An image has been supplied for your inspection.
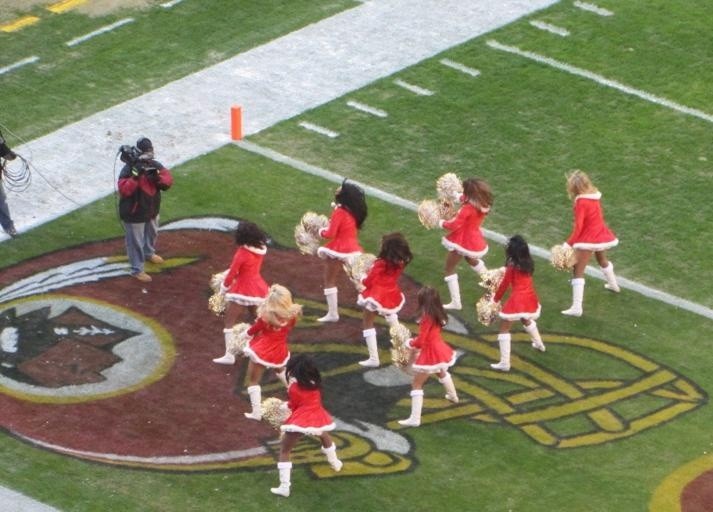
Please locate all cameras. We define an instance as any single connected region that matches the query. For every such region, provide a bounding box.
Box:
[119,144,161,181]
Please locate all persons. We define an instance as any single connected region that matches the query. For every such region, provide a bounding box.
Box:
[233,283,304,422]
[418,171,500,309]
[390,283,462,426]
[263,351,344,499]
[293,177,369,323]
[0,127,18,241]
[478,234,547,372]
[350,230,414,368]
[206,219,276,366]
[114,138,174,284]
[549,168,621,317]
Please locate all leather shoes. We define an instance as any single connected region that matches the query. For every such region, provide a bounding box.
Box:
[131,271,152,282]
[146,254,164,263]
[4,222,16,237]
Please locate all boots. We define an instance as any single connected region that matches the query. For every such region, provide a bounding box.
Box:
[491,334,511,371]
[386,314,400,331]
[399,390,423,427]
[441,273,463,308]
[351,273,368,294]
[244,385,262,421]
[471,259,488,277]
[321,442,343,472]
[523,320,545,352]
[276,367,291,393]
[438,372,458,403]
[318,288,339,322]
[600,262,620,293]
[213,329,235,365]
[359,328,378,366]
[561,278,585,317]
[271,462,292,497]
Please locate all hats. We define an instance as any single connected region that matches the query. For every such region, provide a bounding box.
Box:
[136,137,153,149]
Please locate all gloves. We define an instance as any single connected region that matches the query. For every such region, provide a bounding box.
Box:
[132,163,142,177]
[145,167,160,179]
